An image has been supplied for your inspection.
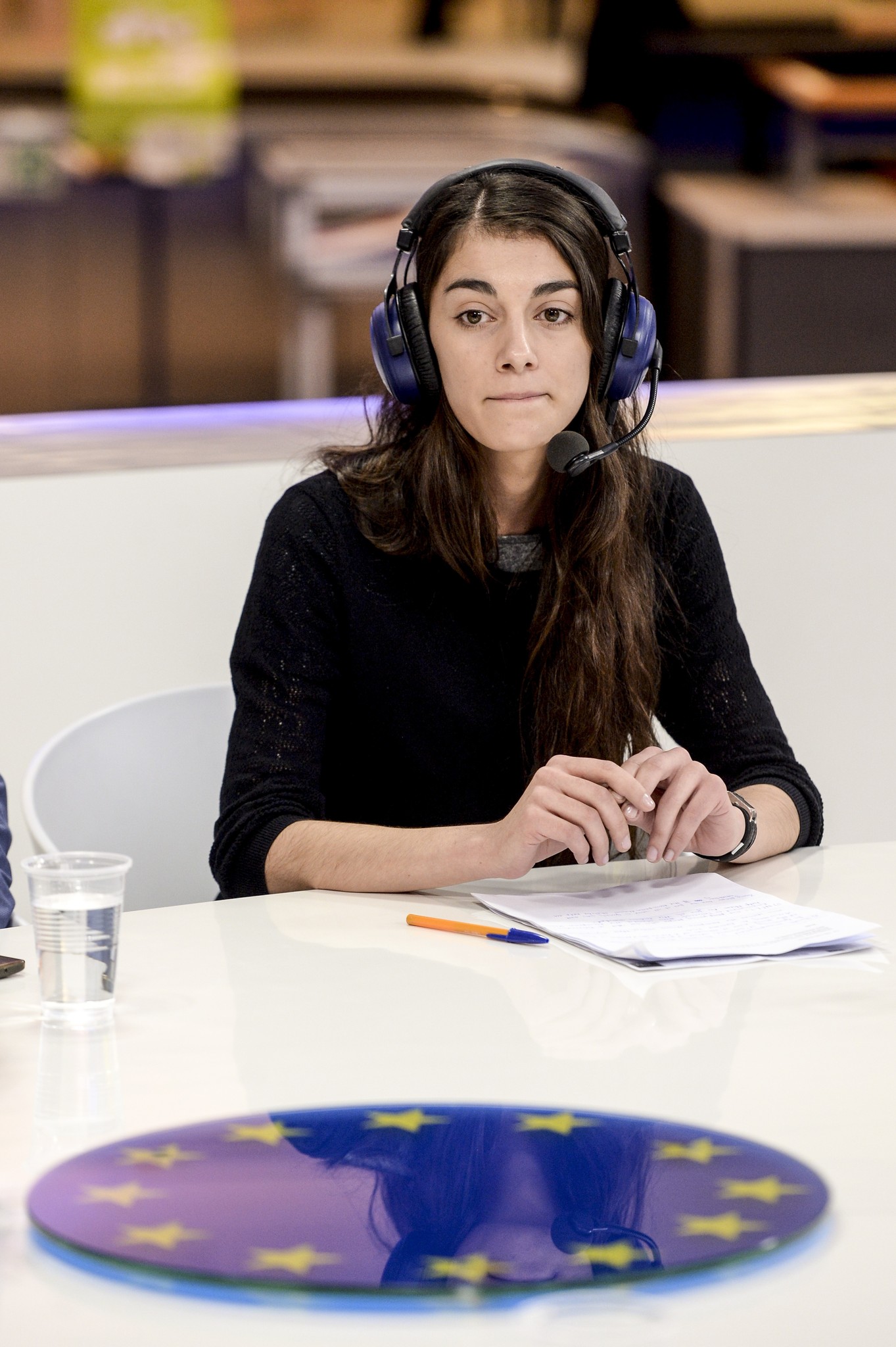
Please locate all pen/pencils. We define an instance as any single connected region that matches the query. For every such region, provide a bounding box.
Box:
[406,913,549,945]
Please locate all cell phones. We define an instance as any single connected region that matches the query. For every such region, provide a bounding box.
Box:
[0,955,25,978]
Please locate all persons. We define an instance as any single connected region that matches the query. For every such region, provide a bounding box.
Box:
[209,159,824,902]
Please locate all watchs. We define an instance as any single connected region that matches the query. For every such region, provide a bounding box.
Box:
[692,791,757,862]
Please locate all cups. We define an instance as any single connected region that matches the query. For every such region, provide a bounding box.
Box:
[19,851,133,1028]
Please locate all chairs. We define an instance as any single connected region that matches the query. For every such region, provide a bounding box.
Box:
[22,680,249,901]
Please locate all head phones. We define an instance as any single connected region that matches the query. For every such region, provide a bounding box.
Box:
[368,161,658,407]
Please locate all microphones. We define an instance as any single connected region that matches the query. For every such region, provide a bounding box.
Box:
[547,340,664,475]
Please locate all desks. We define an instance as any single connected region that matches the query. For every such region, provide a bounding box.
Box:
[660,169,894,381]
[0,839,895,1347]
